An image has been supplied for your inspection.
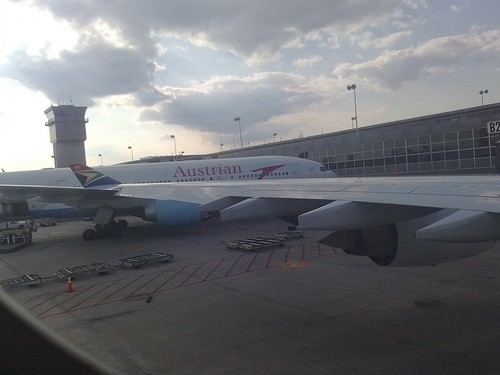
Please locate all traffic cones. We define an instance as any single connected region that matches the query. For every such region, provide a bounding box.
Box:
[65,276,73,293]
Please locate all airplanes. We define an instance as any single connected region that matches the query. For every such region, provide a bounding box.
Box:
[0,155,500,269]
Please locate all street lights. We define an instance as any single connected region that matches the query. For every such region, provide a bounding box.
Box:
[99,154,103,165]
[234,117,242,147]
[347,83,358,128]
[479,89,488,105]
[128,146,134,161]
[351,117,356,129]
[273,133,277,142]
[170,135,176,155]
